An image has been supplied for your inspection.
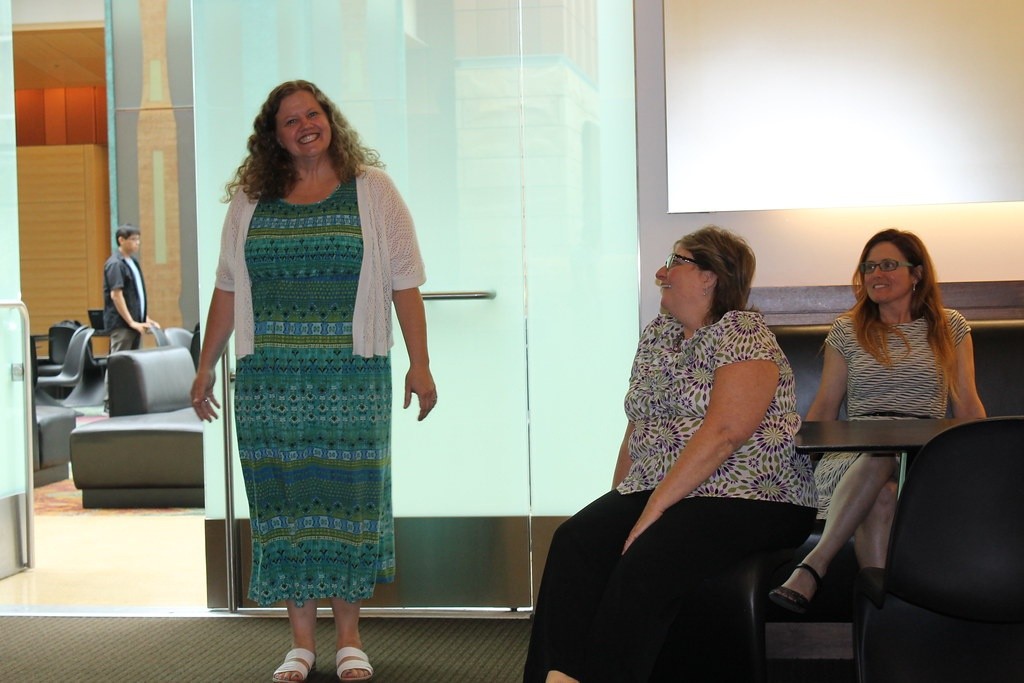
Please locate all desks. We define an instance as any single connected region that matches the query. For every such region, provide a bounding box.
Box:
[794,416,1024,500]
[31,330,110,359]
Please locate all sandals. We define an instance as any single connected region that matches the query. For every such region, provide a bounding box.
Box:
[769,564,823,614]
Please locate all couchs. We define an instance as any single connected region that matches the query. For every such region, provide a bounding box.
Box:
[33,347,205,508]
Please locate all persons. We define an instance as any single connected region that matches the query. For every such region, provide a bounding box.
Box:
[771,228,987,615]
[104,223,160,414]
[521,228,818,683]
[190,80,437,683]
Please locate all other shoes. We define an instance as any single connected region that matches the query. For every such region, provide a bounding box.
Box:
[104,401,109,412]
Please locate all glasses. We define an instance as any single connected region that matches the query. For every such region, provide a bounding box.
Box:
[665,253,697,268]
[859,259,913,274]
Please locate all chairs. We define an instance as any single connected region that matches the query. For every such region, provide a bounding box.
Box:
[29,320,200,415]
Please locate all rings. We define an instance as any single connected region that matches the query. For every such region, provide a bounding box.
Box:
[205,400,208,402]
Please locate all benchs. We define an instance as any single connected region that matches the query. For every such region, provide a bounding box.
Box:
[723,321,1024,682]
[841,415,1024,682]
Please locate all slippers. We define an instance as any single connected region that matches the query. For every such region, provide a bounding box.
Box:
[335,647,374,683]
[272,648,316,683]
